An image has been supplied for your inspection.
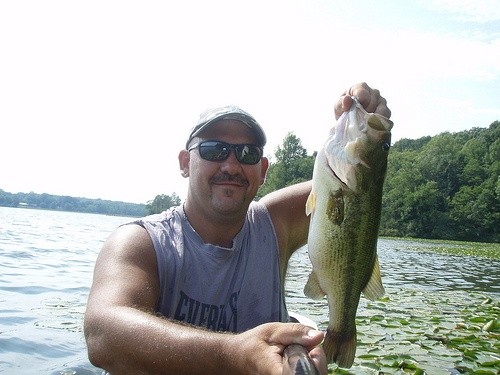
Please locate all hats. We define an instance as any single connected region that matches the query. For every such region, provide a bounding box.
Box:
[185,105,266,150]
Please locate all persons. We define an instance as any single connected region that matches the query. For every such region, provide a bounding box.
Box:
[84,82,391,375]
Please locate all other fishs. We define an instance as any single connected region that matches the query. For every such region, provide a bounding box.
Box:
[303,96,393,368]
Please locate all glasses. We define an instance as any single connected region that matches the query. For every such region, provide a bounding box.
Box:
[189,139,262,165]
